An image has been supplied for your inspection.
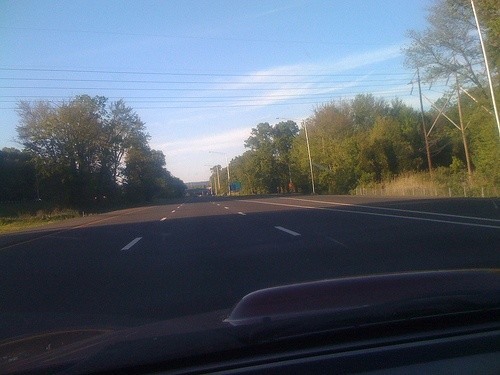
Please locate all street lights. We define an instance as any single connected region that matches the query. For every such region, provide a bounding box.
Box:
[209,151,232,197]
[276,118,316,195]
